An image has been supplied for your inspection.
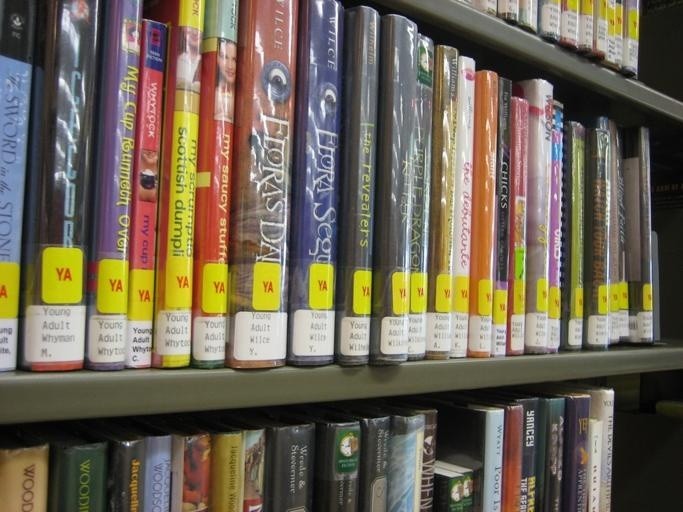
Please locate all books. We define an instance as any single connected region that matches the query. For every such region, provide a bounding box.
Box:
[2,384,615,512]
[1,0,658,374]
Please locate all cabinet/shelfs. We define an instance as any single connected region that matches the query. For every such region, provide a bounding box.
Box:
[0,1,683,512]
